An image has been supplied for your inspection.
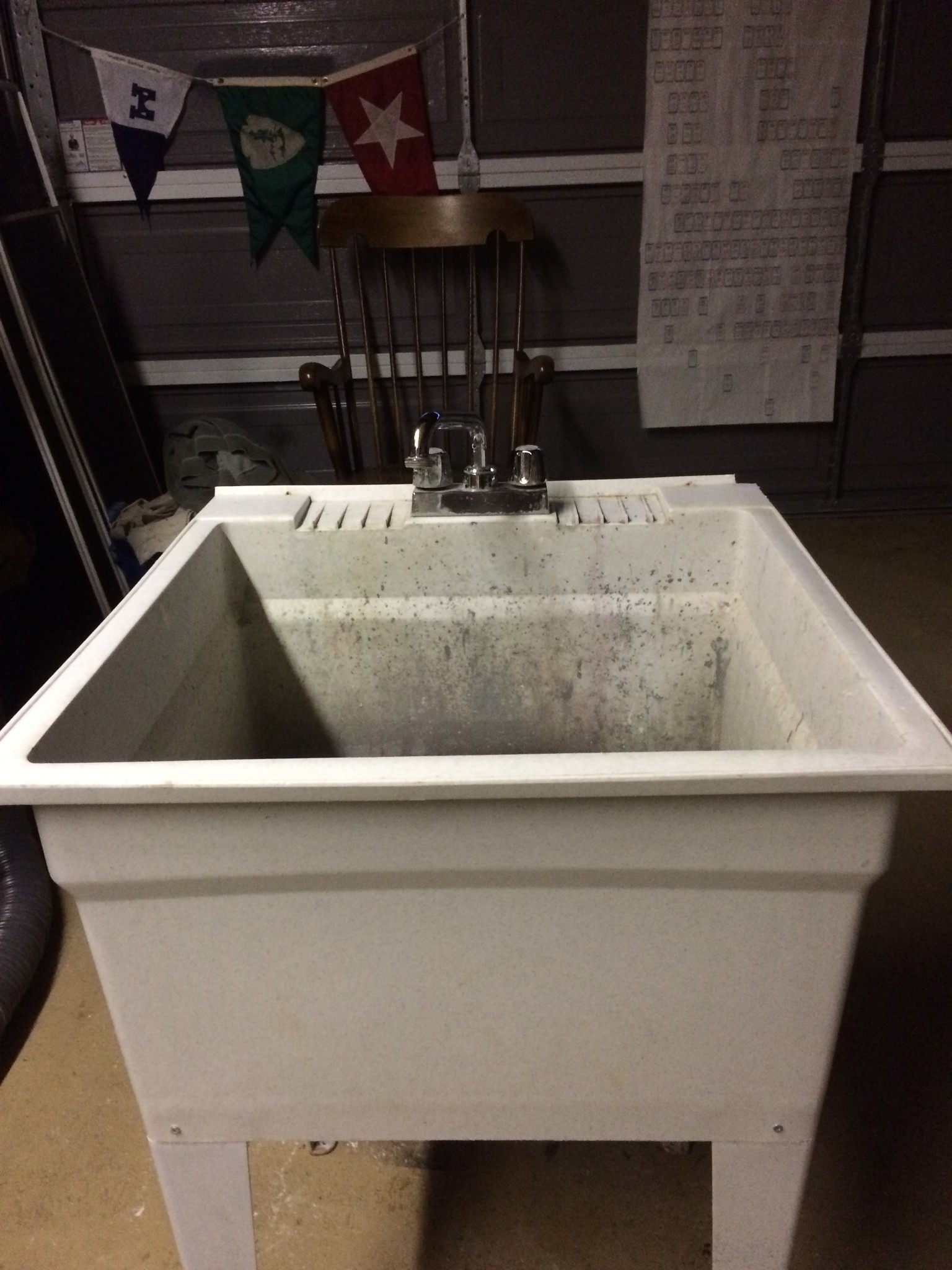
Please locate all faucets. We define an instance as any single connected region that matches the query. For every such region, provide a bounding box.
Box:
[405,410,554,518]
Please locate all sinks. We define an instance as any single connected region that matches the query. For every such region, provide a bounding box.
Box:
[1,477,952,1150]
